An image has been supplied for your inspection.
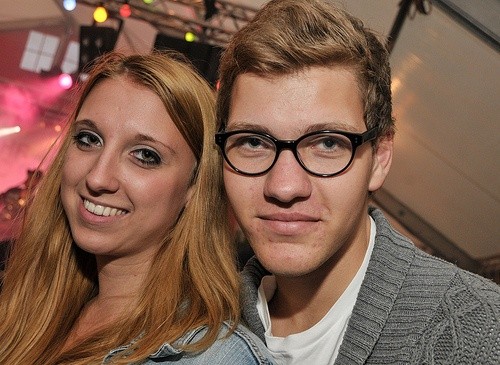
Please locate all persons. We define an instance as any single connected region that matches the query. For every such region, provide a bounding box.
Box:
[214,0,500,365]
[0,52,278,365]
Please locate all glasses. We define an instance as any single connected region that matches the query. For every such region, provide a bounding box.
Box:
[215,121,379,177]
